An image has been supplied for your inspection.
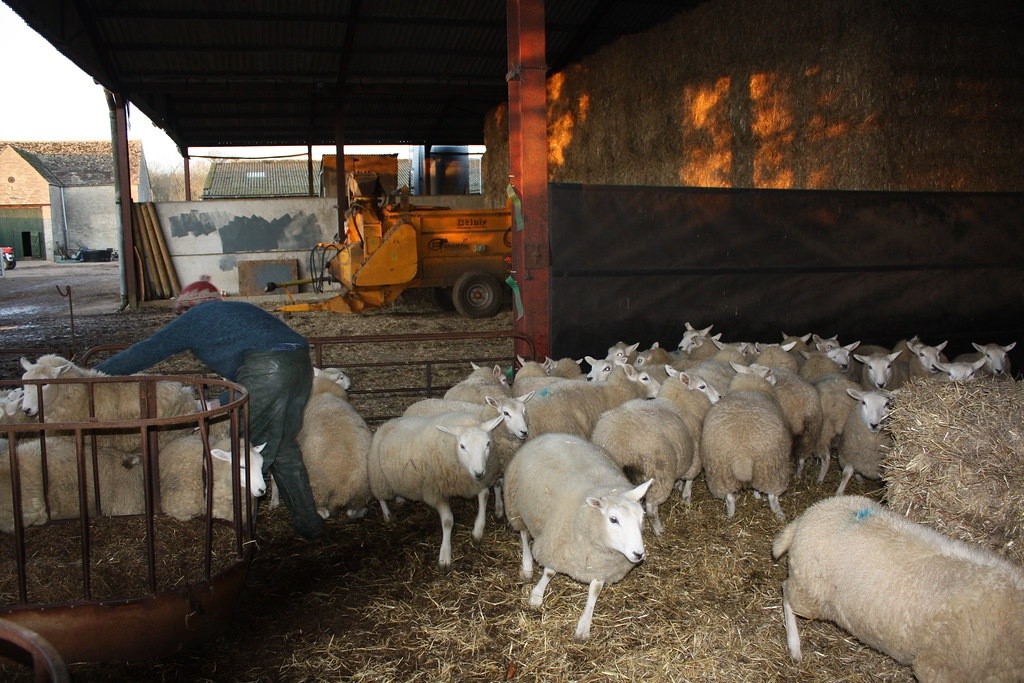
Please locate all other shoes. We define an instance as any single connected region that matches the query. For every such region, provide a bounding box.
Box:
[304,522,326,539]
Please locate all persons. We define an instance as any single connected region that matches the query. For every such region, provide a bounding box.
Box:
[88,281,325,537]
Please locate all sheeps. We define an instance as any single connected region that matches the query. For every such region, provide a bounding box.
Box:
[771,493,1023,682]
[0,320,1023,645]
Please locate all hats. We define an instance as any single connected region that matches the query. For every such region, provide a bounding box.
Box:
[175,280,221,316]
